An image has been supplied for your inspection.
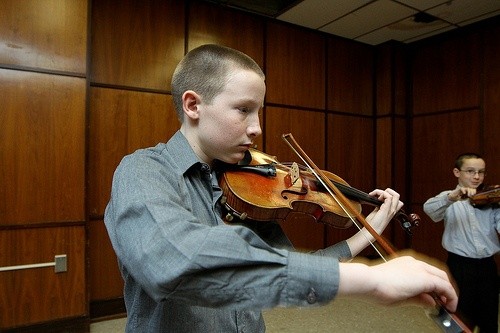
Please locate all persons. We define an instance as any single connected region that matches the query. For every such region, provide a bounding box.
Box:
[103,43,460,333]
[422,152,500,333]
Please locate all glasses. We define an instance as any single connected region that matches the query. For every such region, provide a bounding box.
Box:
[459,169,487,176]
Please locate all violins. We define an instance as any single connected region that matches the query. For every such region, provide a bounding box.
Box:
[470,184,500,206]
[218,147,421,233]
